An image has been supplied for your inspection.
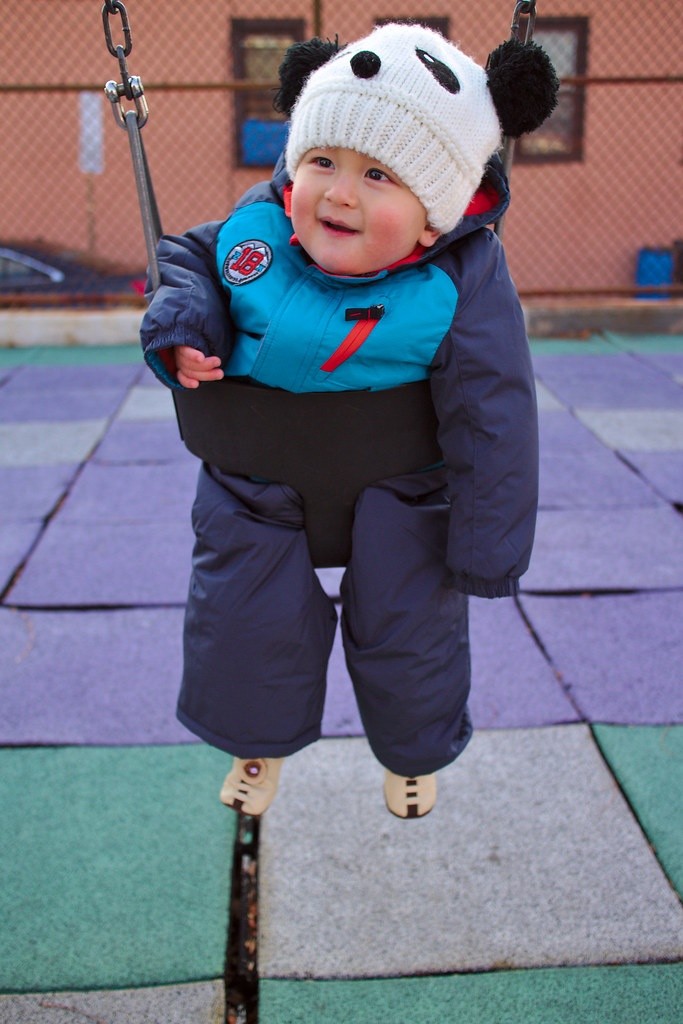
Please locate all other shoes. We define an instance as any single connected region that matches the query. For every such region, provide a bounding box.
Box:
[381,768,437,818]
[219,753,284,816]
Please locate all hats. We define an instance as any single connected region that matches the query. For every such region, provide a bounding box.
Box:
[272,20,562,233]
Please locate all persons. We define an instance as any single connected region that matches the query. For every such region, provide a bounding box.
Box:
[139,24,559,819]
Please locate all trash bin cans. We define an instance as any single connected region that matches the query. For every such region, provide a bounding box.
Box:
[635,247,673,300]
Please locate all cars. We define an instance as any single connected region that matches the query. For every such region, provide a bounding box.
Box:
[0,242,148,298]
